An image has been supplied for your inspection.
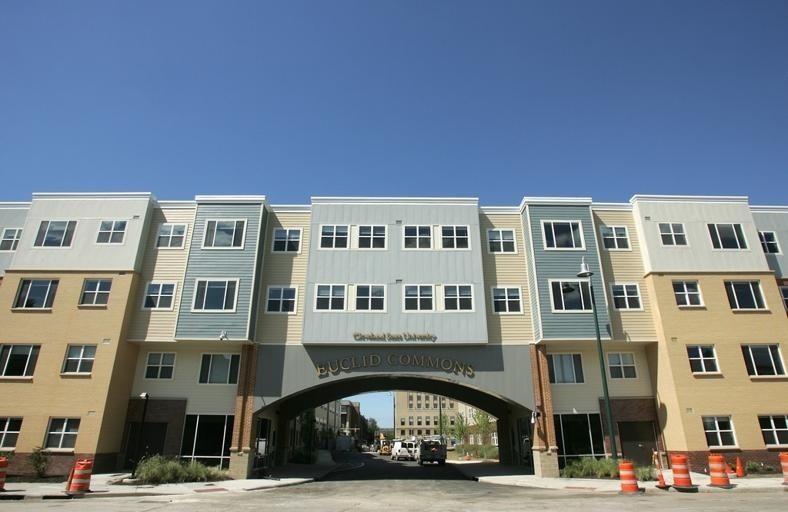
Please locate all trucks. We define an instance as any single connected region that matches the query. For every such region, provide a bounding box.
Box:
[372,437,447,466]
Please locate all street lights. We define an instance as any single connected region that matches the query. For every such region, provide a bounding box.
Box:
[574,251,617,468]
[129,392,149,480]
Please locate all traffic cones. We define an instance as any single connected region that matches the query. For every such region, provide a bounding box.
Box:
[733,457,746,479]
